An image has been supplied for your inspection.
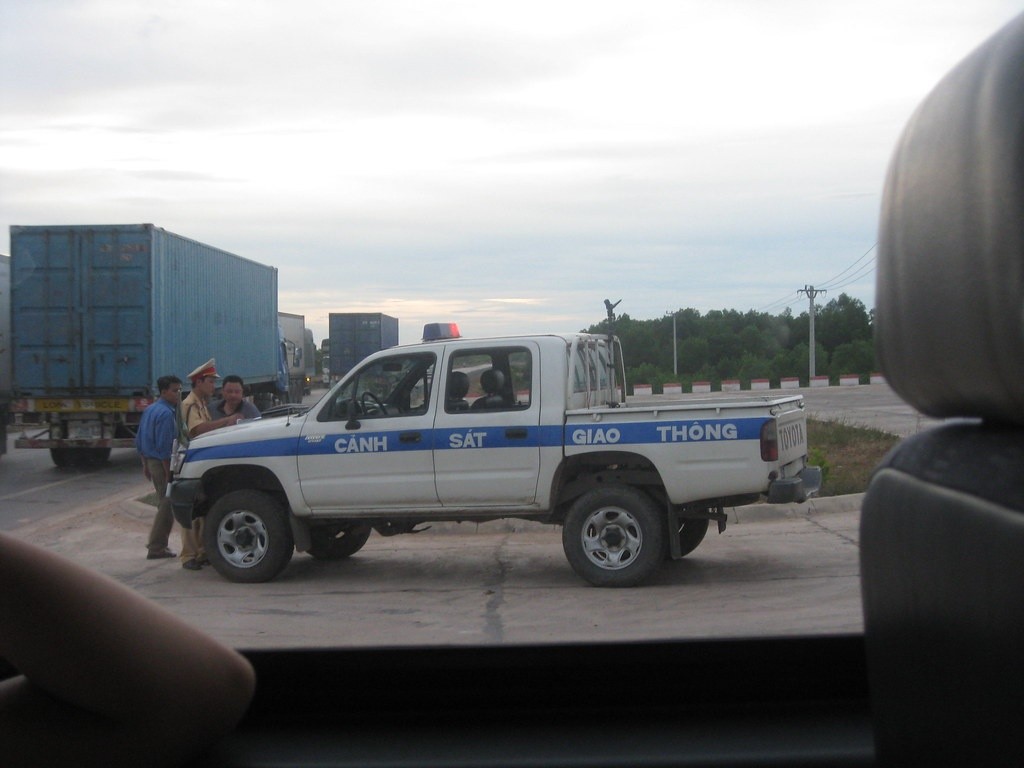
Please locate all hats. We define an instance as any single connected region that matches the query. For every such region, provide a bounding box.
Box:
[187,358,221,383]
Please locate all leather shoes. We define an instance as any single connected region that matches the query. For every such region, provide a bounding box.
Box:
[147,548,177,559]
[183,559,208,570]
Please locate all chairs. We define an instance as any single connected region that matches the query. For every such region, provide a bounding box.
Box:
[471,369,505,409]
[857,8,1024,768]
[447,370,470,412]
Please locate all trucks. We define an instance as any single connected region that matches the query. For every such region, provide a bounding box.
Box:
[9,223,303,472]
[0,254,14,454]
[276,311,317,396]
[328,312,400,391]
[320,338,345,388]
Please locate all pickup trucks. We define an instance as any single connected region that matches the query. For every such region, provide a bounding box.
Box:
[167,319,821,588]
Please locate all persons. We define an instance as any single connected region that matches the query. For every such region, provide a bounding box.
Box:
[208,373,262,424]
[133,374,184,559]
[173,356,227,570]
